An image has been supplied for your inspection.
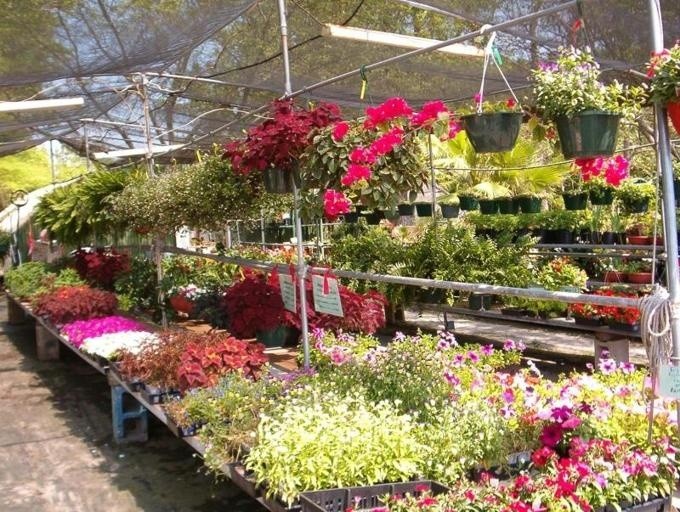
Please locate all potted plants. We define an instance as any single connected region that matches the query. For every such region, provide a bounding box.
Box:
[225,278,290,347]
[601,258,626,282]
[479,197,498,214]
[562,189,588,210]
[103,177,158,233]
[617,183,658,214]
[590,176,616,206]
[415,201,433,217]
[398,200,415,216]
[498,194,518,214]
[162,267,216,316]
[221,95,340,192]
[471,214,625,245]
[627,259,652,284]
[457,189,480,210]
[227,405,418,512]
[513,191,548,213]
[468,270,496,311]
[627,223,650,244]
[438,199,459,218]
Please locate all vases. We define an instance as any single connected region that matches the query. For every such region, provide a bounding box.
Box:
[666,94,680,136]
[459,109,522,155]
[608,318,640,331]
[571,313,605,326]
[550,109,623,158]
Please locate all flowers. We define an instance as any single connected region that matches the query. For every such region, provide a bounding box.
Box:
[471,94,523,113]
[607,291,641,324]
[571,291,606,319]
[530,44,621,117]
[639,39,680,105]
